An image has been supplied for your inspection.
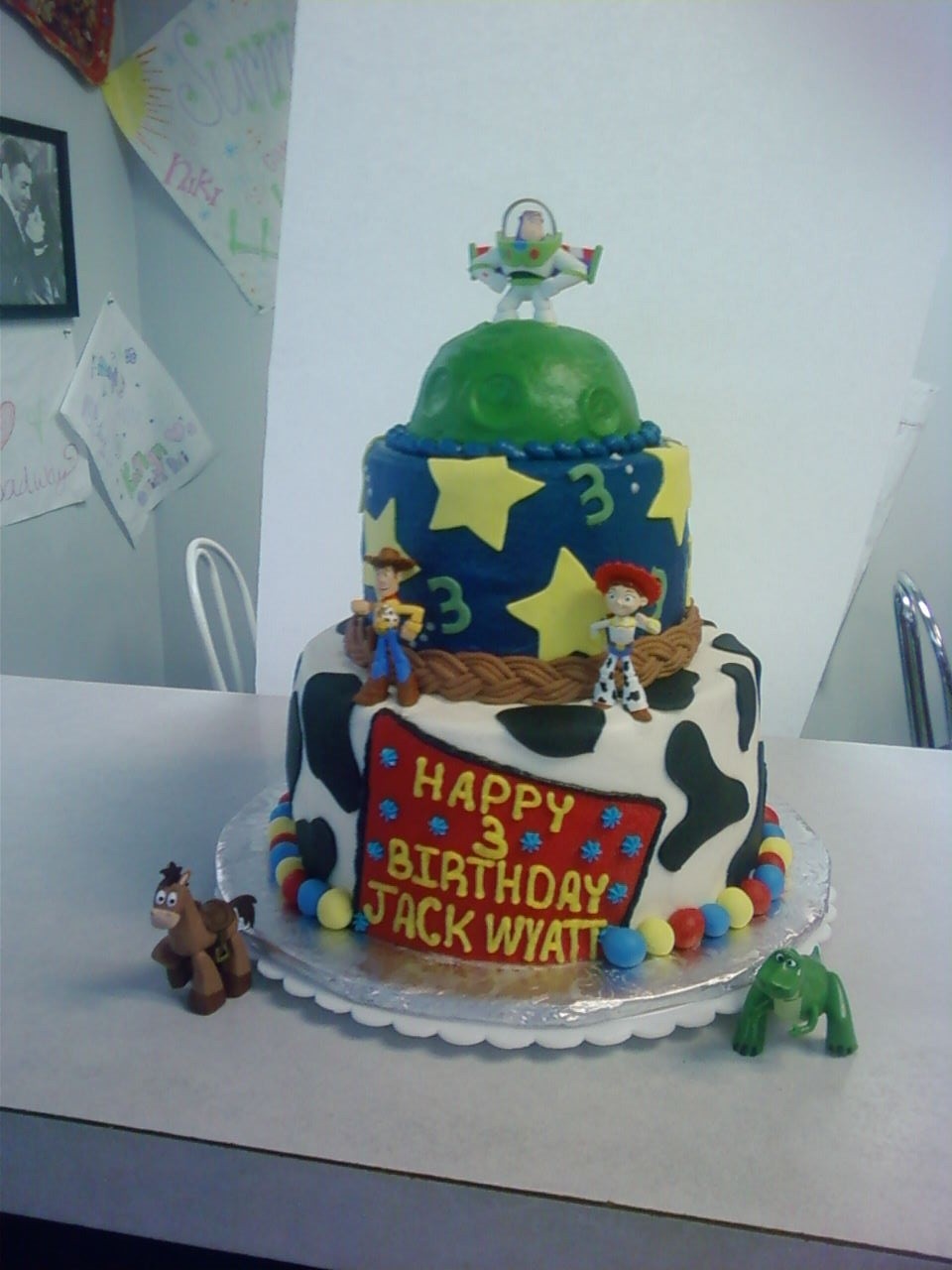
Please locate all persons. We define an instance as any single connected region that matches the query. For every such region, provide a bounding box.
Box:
[350,546,424,705]
[0,136,68,306]
[468,198,604,322]
[588,562,662,722]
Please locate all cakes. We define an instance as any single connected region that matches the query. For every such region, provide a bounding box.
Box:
[261,199,794,967]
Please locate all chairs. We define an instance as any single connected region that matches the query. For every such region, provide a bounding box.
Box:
[890,568,952,756]
[184,535,256,691]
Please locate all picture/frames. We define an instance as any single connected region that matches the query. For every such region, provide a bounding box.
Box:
[0,114,82,321]
